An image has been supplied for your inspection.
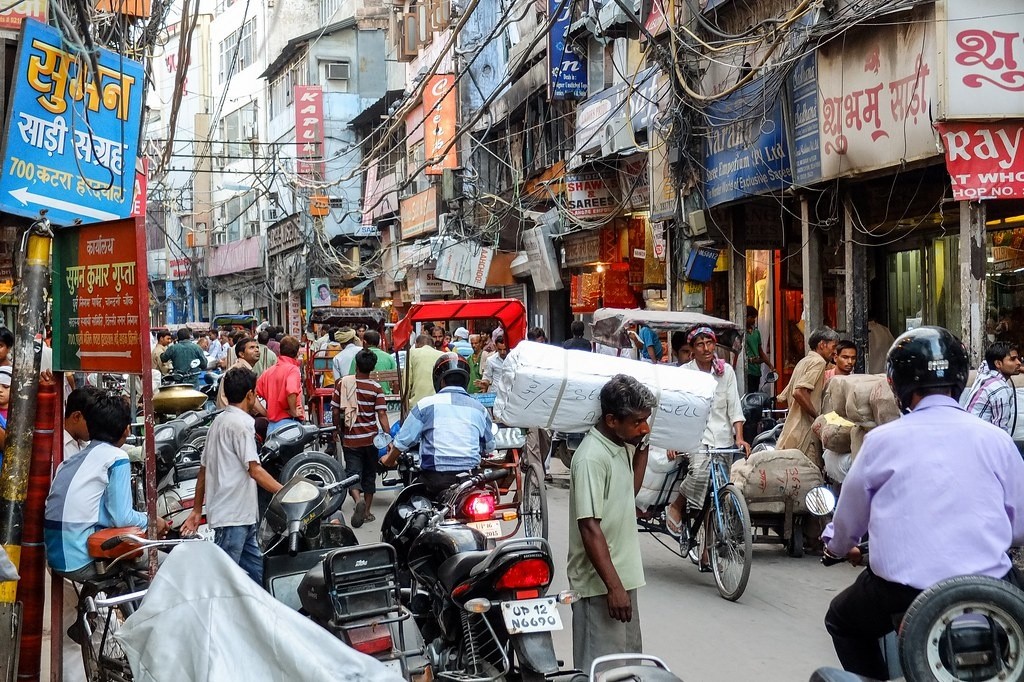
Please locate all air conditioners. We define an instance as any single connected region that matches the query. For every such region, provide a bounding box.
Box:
[599,117,635,156]
[216,157,224,169]
[325,63,350,80]
[218,233,226,245]
[243,125,253,139]
[245,205,257,223]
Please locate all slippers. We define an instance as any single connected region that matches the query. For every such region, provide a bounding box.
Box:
[701,561,714,572]
[664,505,684,537]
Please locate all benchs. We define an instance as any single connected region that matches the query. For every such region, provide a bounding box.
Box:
[307,349,343,371]
[368,369,403,400]
[308,369,334,404]
[468,392,518,428]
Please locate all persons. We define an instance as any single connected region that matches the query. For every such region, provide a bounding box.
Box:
[819,325,1024,681]
[559,306,860,573]
[159,317,306,442]
[0,326,171,647]
[865,310,896,375]
[963,341,1022,435]
[379,318,553,496]
[567,373,657,677]
[178,367,283,585]
[297,323,403,528]
[317,284,331,304]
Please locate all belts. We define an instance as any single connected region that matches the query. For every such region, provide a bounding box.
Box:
[284,416,303,424]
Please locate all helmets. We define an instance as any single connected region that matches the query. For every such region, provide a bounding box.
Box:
[432,351,471,393]
[884,324,969,414]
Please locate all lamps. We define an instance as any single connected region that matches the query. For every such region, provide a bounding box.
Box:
[212,218,222,231]
[262,209,278,222]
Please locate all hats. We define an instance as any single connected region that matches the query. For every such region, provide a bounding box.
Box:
[0,366,12,386]
[335,326,357,343]
[454,327,469,339]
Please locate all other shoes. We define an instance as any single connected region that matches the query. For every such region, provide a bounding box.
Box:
[363,512,376,523]
[350,502,366,528]
[804,545,826,556]
[67,623,80,645]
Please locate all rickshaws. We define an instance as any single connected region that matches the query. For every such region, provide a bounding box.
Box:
[517,422,755,602]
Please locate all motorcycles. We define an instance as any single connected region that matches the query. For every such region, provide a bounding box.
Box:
[800,486,1023,682]
[64,306,792,682]
